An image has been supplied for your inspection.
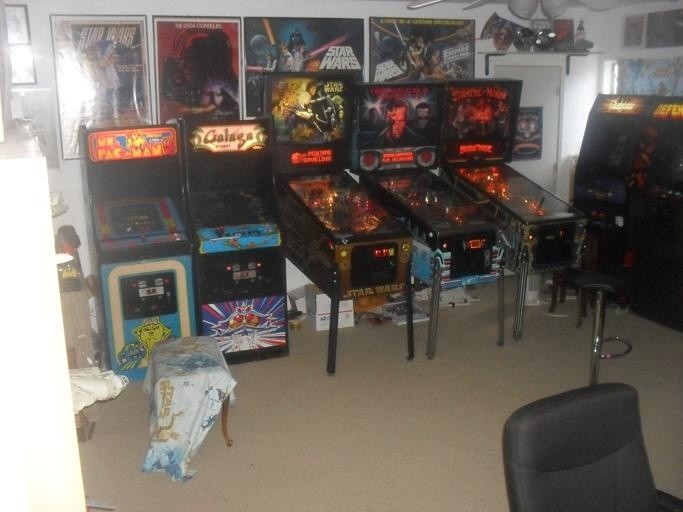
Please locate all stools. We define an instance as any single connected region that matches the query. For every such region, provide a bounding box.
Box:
[562,267,638,384]
[151,335,233,450]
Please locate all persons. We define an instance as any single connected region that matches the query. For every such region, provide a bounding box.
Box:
[363,99,436,149]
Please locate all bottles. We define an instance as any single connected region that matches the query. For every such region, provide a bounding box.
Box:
[574,19,588,51]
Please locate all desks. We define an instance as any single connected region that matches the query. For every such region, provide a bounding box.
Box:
[58,251,96,370]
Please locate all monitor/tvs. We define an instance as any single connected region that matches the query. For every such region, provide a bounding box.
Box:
[583,123,635,178]
[198,192,260,228]
[108,204,164,235]
[662,126,683,190]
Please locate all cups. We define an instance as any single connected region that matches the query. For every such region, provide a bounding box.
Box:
[48,191,61,206]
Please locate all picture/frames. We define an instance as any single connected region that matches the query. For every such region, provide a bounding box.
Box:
[4,3,32,46]
[50,14,152,162]
[9,46,37,85]
[152,15,243,126]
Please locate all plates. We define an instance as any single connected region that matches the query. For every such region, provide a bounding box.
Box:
[532,27,556,48]
[56,253,74,263]
[512,27,536,51]
[491,26,512,51]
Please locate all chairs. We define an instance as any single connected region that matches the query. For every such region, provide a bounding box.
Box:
[502,382,683,511]
[549,226,602,327]
